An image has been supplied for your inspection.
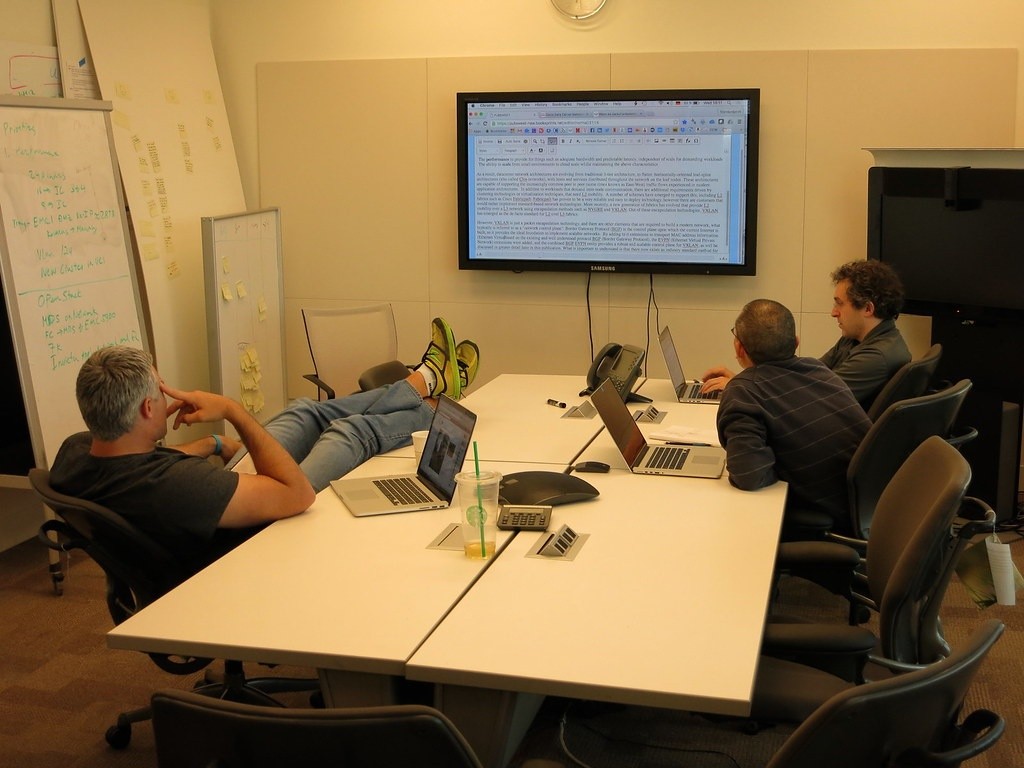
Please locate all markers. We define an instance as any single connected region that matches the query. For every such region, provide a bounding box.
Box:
[547,398,566,408]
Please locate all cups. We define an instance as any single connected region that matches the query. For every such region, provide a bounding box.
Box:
[411,431,430,465]
[454,471,503,559]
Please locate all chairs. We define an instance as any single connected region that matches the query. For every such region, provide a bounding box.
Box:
[554,615,1006,768]
[790,379,975,532]
[300,303,415,409]
[146,686,486,768]
[863,343,944,422]
[765,435,996,682]
[26,463,326,748]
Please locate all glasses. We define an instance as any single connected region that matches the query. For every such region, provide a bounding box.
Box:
[732,327,749,355]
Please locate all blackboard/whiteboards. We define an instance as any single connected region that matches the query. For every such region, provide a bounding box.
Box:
[0,96,147,472]
[201,206,287,442]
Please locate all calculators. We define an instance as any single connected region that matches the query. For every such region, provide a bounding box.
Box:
[497,503,554,530]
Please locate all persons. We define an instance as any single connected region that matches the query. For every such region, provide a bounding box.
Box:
[701,257,911,414]
[717,299,873,532]
[49,318,479,601]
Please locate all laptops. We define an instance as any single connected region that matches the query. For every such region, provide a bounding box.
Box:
[591,377,726,478]
[330,393,477,517]
[657,325,724,404]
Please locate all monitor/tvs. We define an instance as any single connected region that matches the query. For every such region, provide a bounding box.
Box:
[455,87,760,276]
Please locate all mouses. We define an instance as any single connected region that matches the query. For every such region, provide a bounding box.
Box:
[576,461,610,473]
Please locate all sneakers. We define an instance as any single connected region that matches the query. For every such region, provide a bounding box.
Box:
[453,340,480,400]
[422,317,461,403]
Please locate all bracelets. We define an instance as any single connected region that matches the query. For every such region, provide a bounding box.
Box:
[213,435,222,455]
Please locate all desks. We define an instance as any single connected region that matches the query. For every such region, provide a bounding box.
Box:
[102,368,791,768]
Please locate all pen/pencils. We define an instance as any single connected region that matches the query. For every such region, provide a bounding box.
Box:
[665,438,718,447]
